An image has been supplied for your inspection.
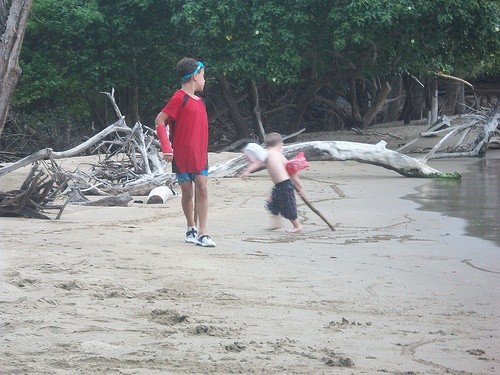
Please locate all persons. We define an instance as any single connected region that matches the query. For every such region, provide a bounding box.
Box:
[240,132,306,234]
[155,58,217,247]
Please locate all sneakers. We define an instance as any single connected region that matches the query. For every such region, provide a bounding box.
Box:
[185,226,198,242]
[197,234,217,246]
